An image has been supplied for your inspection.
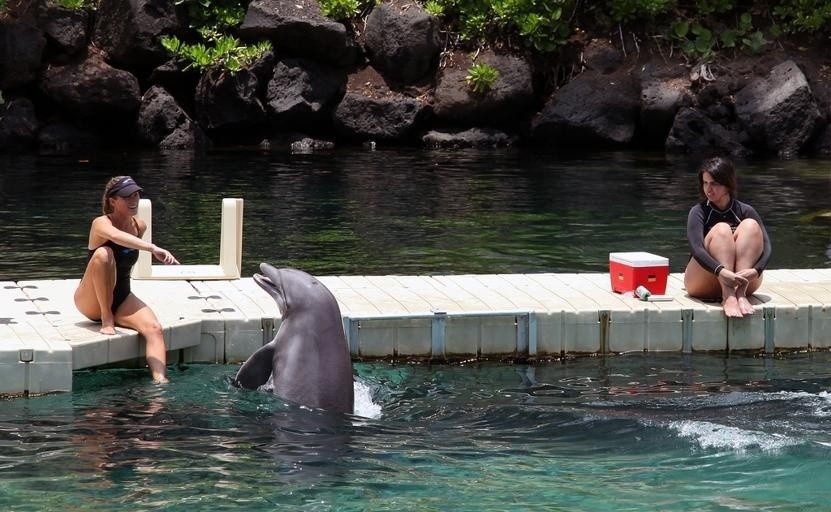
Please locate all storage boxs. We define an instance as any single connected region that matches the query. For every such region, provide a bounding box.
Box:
[608,248,671,295]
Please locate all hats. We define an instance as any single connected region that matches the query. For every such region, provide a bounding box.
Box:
[107,178,143,197]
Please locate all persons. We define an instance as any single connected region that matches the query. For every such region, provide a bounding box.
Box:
[74,175,181,383]
[685,158,772,319]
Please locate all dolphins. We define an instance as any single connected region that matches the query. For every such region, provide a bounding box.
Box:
[233,260,354,420]
